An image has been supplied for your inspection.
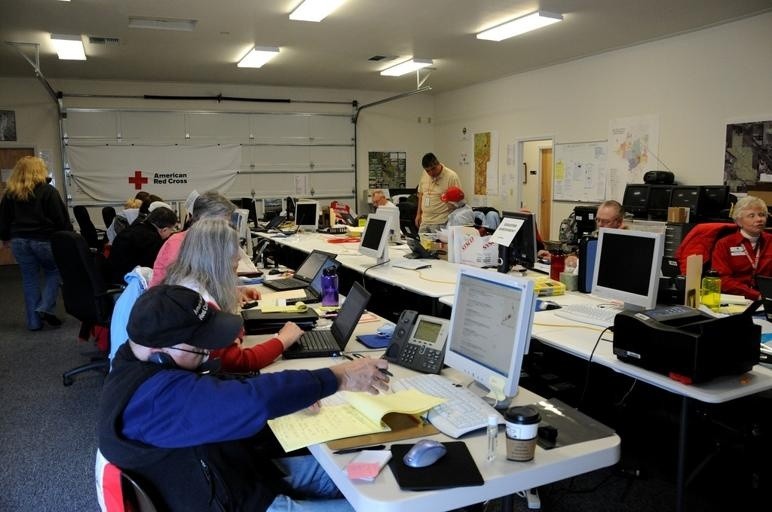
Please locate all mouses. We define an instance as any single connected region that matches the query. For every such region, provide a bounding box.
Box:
[549,300,655,330]
[403,439,447,468]
[400,437,446,468]
[539,301,561,312]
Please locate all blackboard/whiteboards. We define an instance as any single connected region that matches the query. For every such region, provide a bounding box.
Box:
[552,139,608,203]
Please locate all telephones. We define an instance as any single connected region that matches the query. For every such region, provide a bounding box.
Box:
[382,310,450,375]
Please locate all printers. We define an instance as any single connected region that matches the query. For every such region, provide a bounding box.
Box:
[613,300,764,384]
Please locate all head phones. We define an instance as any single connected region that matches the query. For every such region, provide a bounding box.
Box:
[148,352,222,376]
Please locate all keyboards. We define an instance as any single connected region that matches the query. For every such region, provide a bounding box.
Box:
[385,255,432,273]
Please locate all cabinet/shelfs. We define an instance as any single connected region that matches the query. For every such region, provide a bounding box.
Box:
[621,183,730,259]
[389,187,417,199]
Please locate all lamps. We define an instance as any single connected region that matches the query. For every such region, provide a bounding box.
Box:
[51,33,89,63]
[236,45,281,70]
[287,0,335,23]
[380,58,434,78]
[473,10,564,43]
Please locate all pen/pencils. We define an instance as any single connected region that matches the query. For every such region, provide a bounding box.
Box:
[333,445,386,455]
[353,354,393,377]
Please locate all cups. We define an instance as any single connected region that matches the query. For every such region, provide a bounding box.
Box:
[505,406,539,462]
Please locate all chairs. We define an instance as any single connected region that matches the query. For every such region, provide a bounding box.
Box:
[473,206,500,237]
[102,206,116,228]
[72,204,109,258]
[50,229,126,387]
[95,448,156,512]
[395,202,419,237]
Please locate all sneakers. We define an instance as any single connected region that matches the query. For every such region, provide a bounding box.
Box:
[37,311,63,327]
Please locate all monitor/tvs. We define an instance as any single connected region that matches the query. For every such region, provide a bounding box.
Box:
[496,211,537,274]
[358,212,397,265]
[439,264,536,408]
[293,202,319,228]
[590,225,662,311]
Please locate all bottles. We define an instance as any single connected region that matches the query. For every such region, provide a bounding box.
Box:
[701,268,722,314]
[548,247,564,281]
[319,267,341,307]
[486,413,500,462]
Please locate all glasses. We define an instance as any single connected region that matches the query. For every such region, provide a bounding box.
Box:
[161,346,211,364]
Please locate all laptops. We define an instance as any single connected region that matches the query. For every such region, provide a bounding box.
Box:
[259,249,338,292]
[273,280,371,360]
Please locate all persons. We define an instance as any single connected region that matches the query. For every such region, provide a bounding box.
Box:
[0,155,75,331]
[537,199,627,260]
[711,196,772,301]
[415,152,463,235]
[440,187,475,227]
[102,190,304,374]
[97,284,388,512]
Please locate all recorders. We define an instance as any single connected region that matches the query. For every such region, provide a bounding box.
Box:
[644,171,674,185]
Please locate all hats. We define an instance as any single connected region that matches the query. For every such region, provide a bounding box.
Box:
[127,284,242,349]
[440,186,465,202]
